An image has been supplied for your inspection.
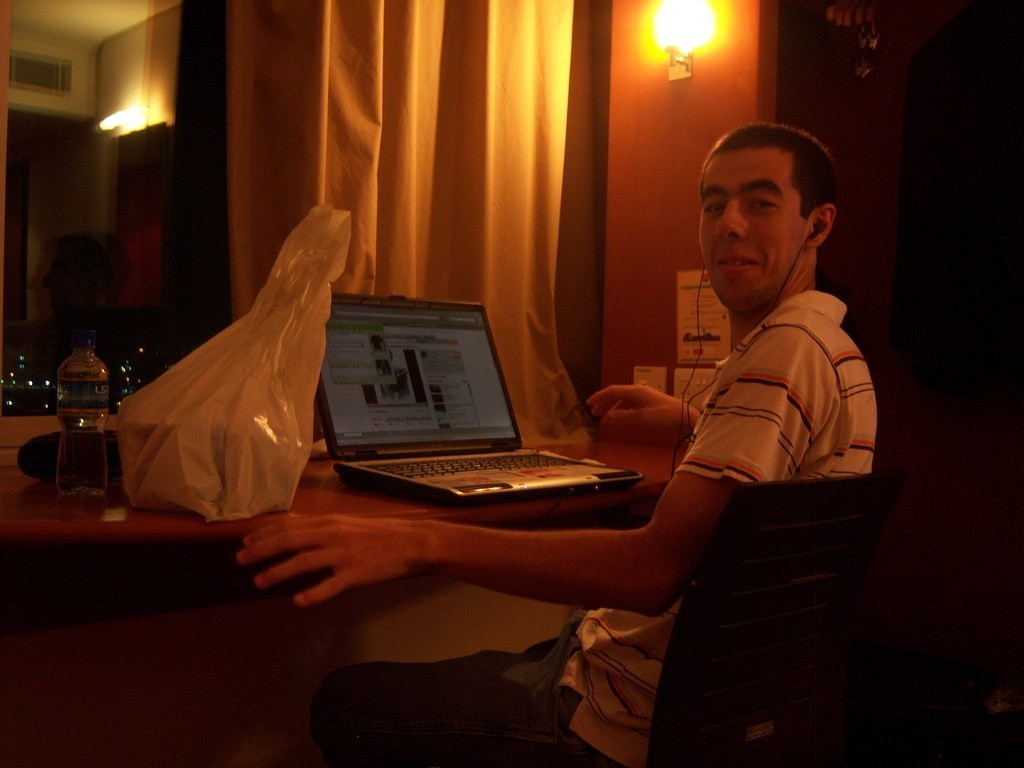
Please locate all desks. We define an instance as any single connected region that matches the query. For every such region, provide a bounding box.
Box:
[1,463,671,551]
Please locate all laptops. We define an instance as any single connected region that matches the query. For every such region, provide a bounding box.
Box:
[314,291,645,508]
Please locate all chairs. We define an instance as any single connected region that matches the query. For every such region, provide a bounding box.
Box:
[646,468,910,768]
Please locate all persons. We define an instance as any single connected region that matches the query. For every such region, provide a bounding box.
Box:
[234,120,878,768]
[28,229,136,320]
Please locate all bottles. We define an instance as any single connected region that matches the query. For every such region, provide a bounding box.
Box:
[56,330,109,495]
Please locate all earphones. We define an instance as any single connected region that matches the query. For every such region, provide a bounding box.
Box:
[809,223,819,240]
